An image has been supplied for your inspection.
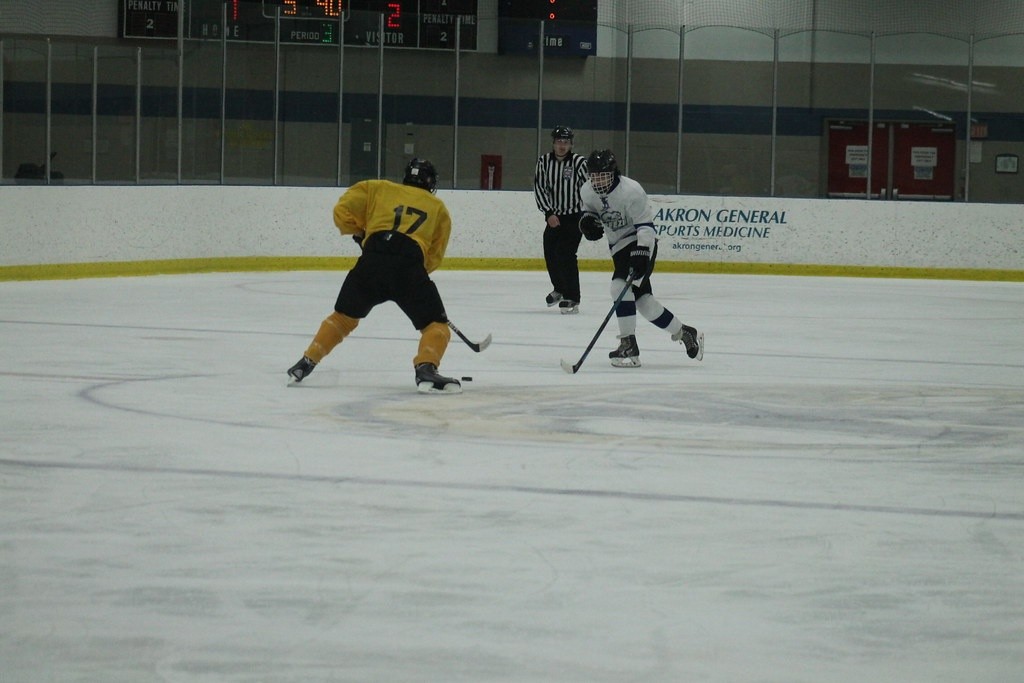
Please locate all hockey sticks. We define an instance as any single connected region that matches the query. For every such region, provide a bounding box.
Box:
[446,318,494,354]
[559,270,637,375]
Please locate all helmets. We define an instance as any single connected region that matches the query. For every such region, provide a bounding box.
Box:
[403,158,438,193]
[587,149,617,194]
[551,126,574,142]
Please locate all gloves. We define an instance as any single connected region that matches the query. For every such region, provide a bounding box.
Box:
[579,215,605,241]
[352,233,364,247]
[628,246,651,279]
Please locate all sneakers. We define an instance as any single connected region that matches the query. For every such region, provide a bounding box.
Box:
[609,335,641,367]
[415,362,462,394]
[287,356,316,387]
[672,325,705,361]
[546,290,562,307]
[559,299,580,314]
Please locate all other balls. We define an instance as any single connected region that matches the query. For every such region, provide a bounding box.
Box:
[461,376,472,381]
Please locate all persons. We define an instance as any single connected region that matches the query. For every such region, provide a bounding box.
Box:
[580,148,705,368]
[534,125,590,314]
[287,158,463,394]
